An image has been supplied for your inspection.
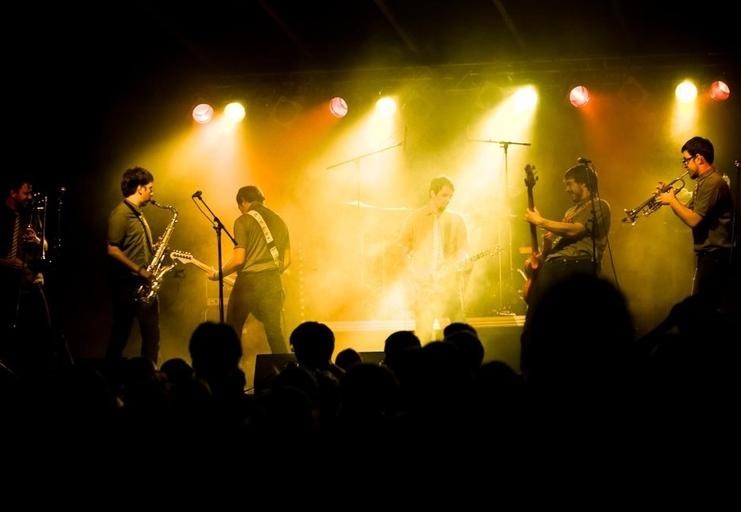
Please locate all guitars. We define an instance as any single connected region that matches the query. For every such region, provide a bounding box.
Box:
[409,244,503,302]
[169,248,286,321]
[522,165,552,304]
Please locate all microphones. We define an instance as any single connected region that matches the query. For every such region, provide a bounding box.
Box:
[192,190,202,199]
[401,125,408,150]
[577,157,593,164]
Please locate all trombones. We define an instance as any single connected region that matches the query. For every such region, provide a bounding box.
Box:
[20,192,48,293]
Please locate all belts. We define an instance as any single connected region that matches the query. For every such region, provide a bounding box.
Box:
[8,211,21,259]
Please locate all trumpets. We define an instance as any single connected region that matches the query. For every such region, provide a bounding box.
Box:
[623,172,688,226]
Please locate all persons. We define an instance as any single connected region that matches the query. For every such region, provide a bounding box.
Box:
[528,165,610,275]
[655,137,740,295]
[0,271,740,512]
[106,167,170,364]
[0,178,48,329]
[209,186,289,355]
[387,178,472,347]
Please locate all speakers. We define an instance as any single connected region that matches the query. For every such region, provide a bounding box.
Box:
[254,354,298,393]
[359,352,385,366]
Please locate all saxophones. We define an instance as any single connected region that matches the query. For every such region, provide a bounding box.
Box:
[133,196,178,302]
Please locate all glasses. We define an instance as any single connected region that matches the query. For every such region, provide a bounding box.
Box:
[681,155,696,168]
